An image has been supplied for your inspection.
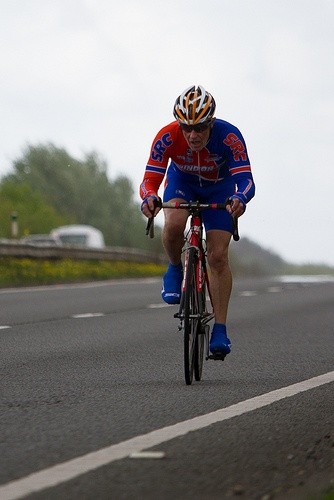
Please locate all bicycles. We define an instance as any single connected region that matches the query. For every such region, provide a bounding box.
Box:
[144,197,241,387]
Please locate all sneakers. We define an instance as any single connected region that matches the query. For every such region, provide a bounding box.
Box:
[161,277,181,303]
[210,333,231,354]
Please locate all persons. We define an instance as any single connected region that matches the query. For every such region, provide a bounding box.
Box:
[139,86,254,354]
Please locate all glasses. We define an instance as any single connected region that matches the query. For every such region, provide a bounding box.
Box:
[180,118,212,133]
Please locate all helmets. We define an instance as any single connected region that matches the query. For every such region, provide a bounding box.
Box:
[173,86,215,125]
